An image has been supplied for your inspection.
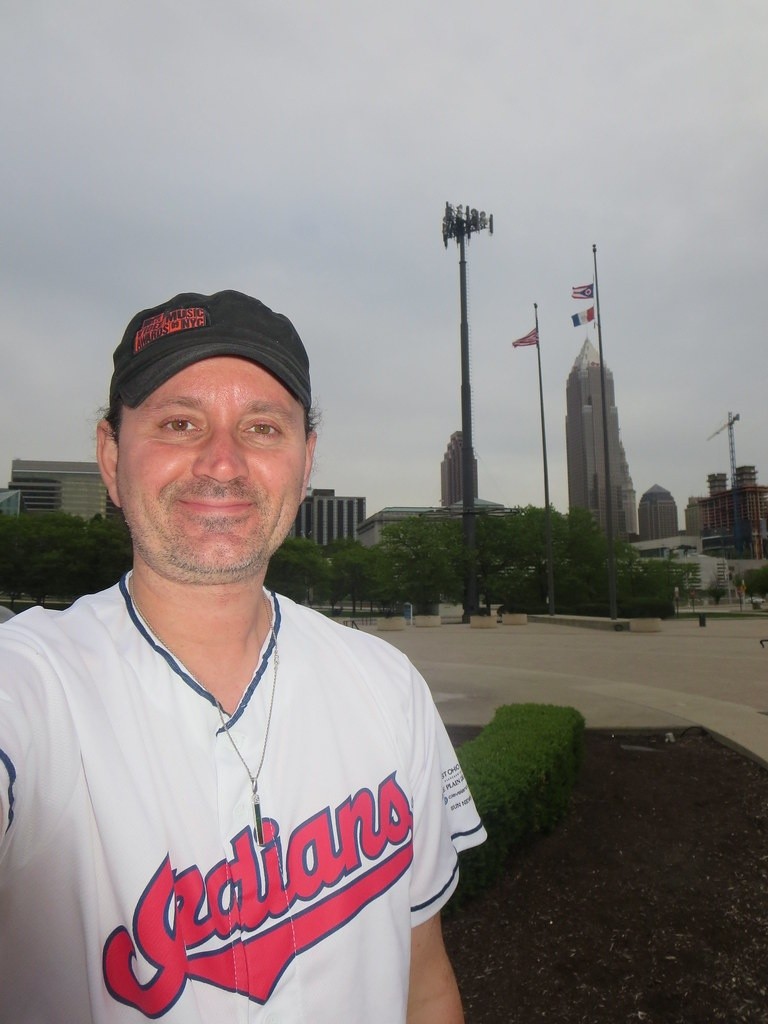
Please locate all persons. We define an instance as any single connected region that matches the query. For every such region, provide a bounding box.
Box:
[0,288,487,1024]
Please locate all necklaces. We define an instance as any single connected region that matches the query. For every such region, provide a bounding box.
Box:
[129,578,279,845]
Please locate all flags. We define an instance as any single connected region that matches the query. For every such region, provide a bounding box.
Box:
[571,306,594,328]
[572,283,594,299]
[512,328,537,349]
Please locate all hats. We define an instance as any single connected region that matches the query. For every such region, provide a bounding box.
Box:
[108,291,311,433]
